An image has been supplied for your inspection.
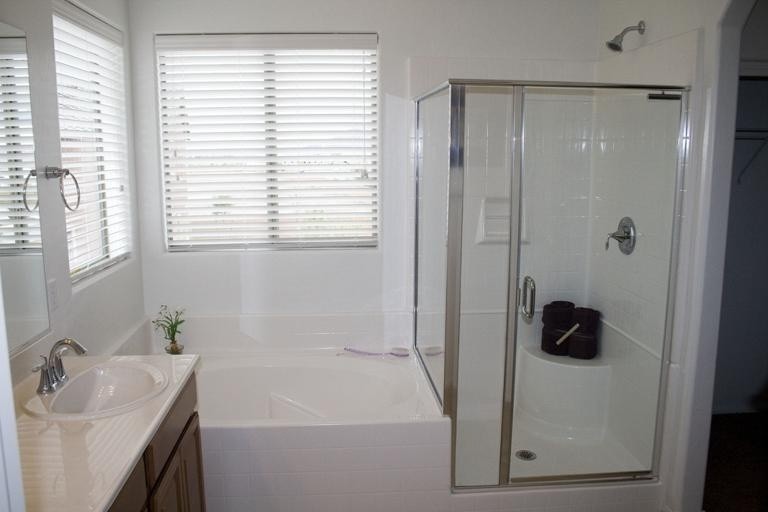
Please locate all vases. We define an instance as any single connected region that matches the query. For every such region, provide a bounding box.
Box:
[162,343,184,356]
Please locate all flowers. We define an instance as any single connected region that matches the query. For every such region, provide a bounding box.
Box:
[151,304,186,354]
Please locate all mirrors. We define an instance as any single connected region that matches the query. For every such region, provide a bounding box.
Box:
[0,22,54,358]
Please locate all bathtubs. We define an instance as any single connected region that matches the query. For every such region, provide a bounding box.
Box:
[194,352,451,429]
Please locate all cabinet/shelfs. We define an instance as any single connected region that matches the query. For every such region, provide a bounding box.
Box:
[108,370,208,512]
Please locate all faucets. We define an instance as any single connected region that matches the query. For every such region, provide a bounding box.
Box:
[47,337,88,387]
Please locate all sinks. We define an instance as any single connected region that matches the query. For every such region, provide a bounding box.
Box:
[21,357,169,422]
